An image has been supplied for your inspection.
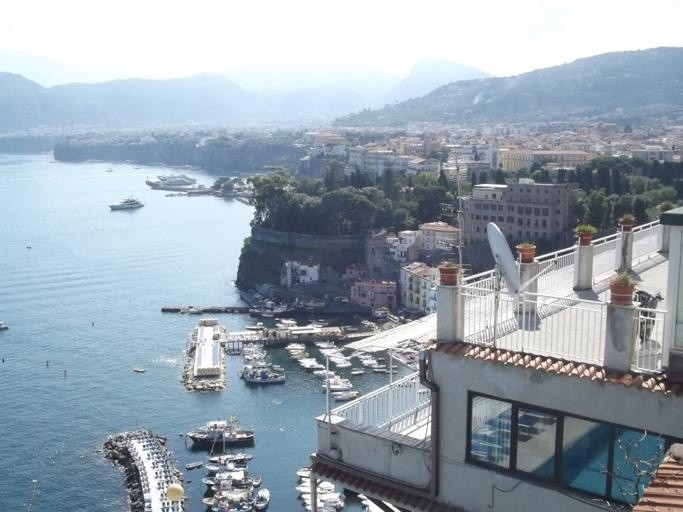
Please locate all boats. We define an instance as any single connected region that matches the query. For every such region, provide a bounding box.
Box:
[186,462,202,470]
[109,196,146,210]
[188,416,256,445]
[299,466,346,512]
[342,325,359,333]
[245,313,330,332]
[286,342,399,401]
[240,342,286,383]
[145,173,201,190]
[206,452,270,512]
[133,367,144,372]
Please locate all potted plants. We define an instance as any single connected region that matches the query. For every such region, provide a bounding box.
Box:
[610,272,636,303]
[618,212,636,232]
[439,259,459,287]
[656,201,677,212]
[573,223,598,247]
[514,240,537,264]
